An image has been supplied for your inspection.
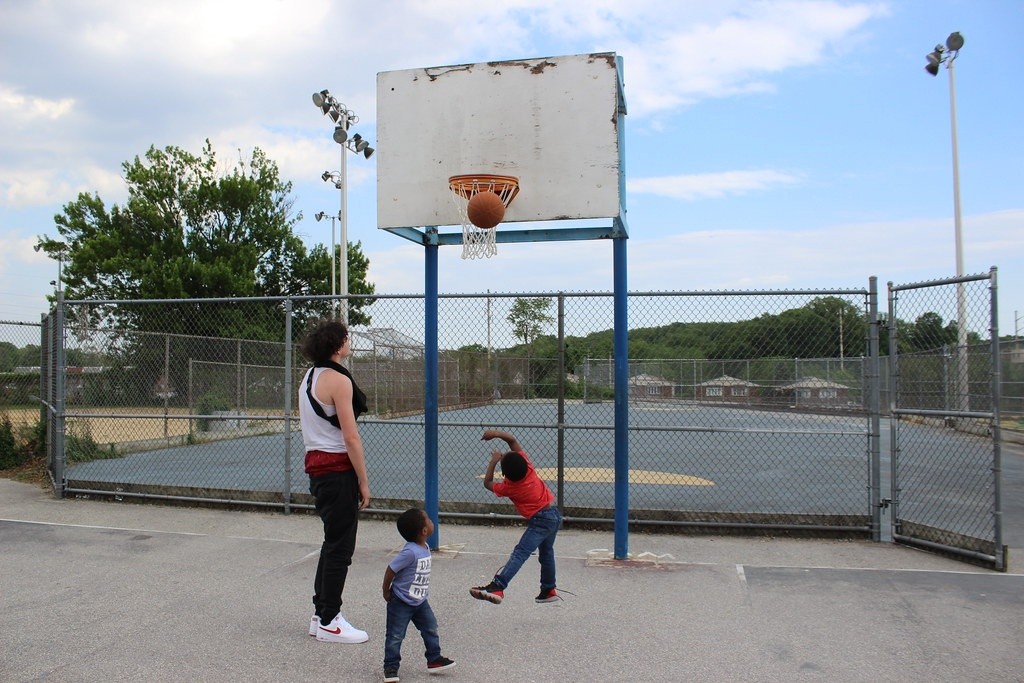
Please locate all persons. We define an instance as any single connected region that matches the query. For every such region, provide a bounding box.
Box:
[382,506,457,683]
[488,386,501,400]
[468,430,563,603]
[298,320,370,644]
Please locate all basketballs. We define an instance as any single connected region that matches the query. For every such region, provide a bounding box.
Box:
[466,191,506,229]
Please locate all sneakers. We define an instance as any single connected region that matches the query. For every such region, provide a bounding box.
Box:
[427,656,456,673]
[316,611,368,644]
[470,566,504,604]
[309,610,321,635]
[384,669,400,682]
[535,587,578,603]
